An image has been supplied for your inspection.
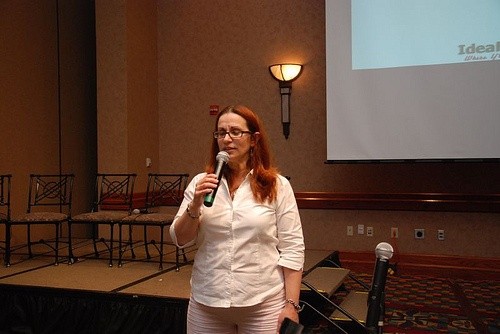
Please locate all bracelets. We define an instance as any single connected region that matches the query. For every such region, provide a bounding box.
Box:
[187,204,201,220]
[286,298,301,313]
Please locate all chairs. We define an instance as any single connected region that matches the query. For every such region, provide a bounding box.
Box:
[9,174,74,266]
[69,174,137,267]
[0,173,12,267]
[118,173,190,271]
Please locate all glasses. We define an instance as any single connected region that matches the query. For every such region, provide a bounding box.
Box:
[213,130,254,139]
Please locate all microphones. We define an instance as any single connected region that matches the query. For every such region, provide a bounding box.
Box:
[365,241,394,334]
[204,151,229,208]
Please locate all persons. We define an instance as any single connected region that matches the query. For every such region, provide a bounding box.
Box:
[168,104,306,334]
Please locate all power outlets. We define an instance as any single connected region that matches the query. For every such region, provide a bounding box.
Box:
[391,228,398,238]
[438,230,444,241]
[358,225,364,234]
[415,229,424,239]
[367,227,373,237]
[347,226,354,236]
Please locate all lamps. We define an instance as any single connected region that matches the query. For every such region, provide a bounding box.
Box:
[269,63,304,141]
